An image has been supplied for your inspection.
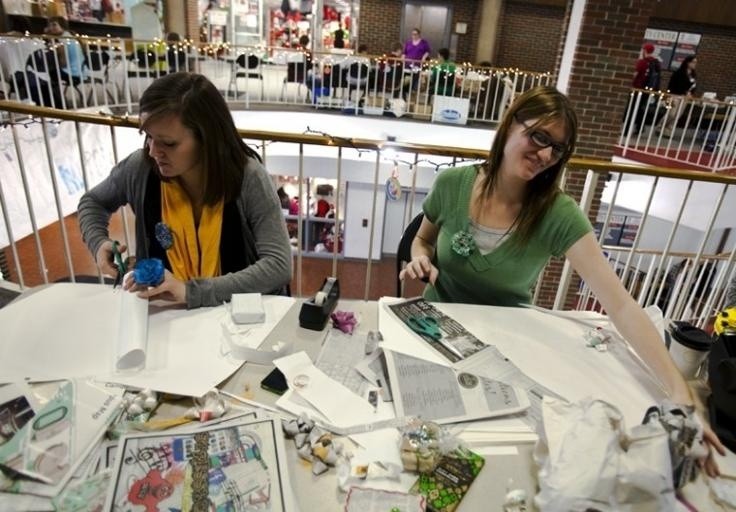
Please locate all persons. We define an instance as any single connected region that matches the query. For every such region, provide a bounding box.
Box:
[156,31,186,77]
[76,71,294,310]
[401,28,431,71]
[47,16,86,84]
[396,85,727,479]
[272,181,340,254]
[344,43,495,119]
[288,35,312,83]
[622,44,660,136]
[655,54,698,138]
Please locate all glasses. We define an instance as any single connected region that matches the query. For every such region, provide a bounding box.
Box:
[521,121,568,159]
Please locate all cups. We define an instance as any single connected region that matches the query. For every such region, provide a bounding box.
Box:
[669,325,713,379]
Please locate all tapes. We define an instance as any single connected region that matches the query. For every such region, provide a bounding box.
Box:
[315,277,336,306]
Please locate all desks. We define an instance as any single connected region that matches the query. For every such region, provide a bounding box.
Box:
[1,282,736,512]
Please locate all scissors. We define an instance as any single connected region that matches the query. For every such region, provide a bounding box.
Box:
[112,240,129,289]
[406,315,465,360]
